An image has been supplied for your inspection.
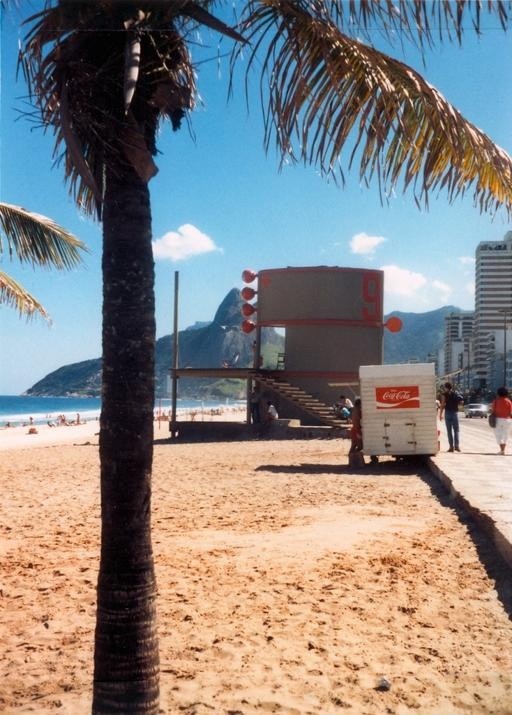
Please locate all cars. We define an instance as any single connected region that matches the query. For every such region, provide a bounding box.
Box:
[464,403,488,419]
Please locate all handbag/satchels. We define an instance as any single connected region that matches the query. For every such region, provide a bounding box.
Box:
[488,413,495,427]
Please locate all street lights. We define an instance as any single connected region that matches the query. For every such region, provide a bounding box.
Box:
[496,307,512,391]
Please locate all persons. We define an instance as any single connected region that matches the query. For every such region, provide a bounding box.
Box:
[76,413,80,425]
[47,421,56,428]
[436,399,441,413]
[264,401,279,425]
[439,382,461,452]
[29,416,33,425]
[335,395,354,421]
[491,387,512,454]
[252,340,256,369]
[249,386,261,424]
[347,398,363,457]
